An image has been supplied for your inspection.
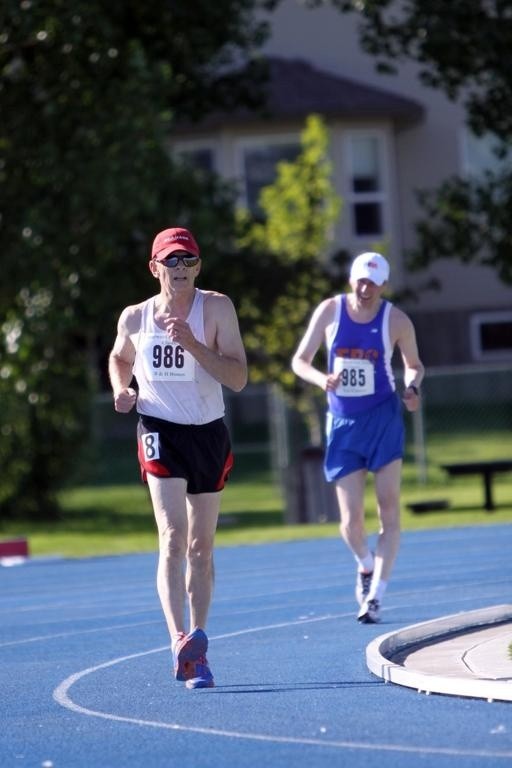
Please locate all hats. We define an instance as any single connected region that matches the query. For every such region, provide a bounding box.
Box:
[152,227,199,262]
[350,252,389,287]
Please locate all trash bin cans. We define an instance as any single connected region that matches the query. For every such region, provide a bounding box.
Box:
[283,447,339,523]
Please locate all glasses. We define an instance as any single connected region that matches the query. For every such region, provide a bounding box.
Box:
[158,255,199,267]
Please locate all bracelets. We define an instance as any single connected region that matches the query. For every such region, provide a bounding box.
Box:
[407,385,418,396]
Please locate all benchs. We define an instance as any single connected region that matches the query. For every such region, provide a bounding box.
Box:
[441,458,512,511]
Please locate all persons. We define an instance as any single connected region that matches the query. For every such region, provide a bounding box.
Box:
[108,225,251,689]
[290,251,425,623]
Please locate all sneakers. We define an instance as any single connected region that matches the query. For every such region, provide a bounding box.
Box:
[187,656,212,689]
[356,553,375,604]
[173,628,208,680]
[357,599,381,624]
